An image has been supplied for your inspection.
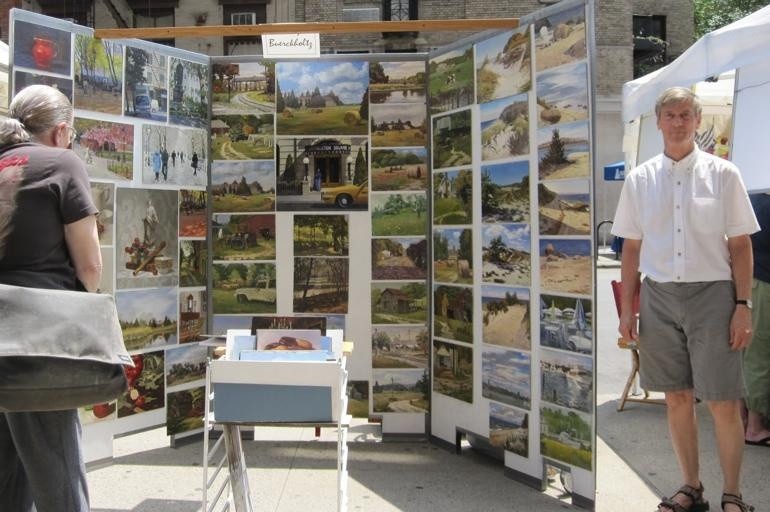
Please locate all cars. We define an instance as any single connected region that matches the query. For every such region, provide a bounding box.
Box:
[134,94,152,118]
[320,177,370,211]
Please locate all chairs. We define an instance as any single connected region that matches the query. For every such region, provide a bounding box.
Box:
[610,272,667,412]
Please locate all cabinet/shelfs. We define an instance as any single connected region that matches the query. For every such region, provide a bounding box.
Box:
[201,341,354,512]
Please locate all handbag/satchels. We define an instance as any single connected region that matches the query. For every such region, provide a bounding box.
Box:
[1,283,137,416]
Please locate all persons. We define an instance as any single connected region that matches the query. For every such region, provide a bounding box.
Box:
[611,86,763,512]
[124,236,149,262]
[411,203,421,216]
[144,198,159,243]
[438,172,451,198]
[84,143,94,164]
[314,168,322,191]
[0,83,104,512]
[460,176,472,205]
[152,148,199,182]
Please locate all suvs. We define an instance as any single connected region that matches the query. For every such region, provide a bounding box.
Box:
[235,280,276,306]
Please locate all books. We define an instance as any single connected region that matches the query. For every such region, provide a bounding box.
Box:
[229,316,332,361]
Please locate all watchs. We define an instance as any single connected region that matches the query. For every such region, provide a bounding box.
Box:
[735,298,753,310]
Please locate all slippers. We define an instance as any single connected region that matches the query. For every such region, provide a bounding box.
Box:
[745,436,770,448]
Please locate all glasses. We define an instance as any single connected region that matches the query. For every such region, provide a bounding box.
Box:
[68,126,77,143]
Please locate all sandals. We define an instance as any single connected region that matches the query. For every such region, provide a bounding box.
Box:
[720,492,755,512]
[655,480,710,512]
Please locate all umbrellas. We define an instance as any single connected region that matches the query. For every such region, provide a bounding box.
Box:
[540,294,591,335]
